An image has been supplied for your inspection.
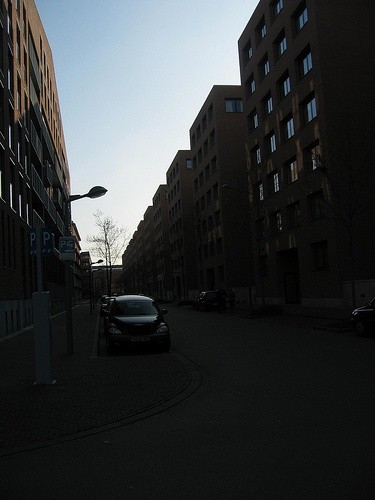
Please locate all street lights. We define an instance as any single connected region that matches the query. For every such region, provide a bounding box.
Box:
[89,260,103,310]
[63,185,108,356]
[220,183,266,310]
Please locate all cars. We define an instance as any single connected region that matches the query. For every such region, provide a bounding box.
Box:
[350,296,375,338]
[104,295,171,354]
[100,294,117,316]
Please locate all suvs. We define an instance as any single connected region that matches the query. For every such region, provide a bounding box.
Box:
[192,289,226,312]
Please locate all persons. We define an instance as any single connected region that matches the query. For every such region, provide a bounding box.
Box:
[218,287,228,314]
[202,287,207,291]
[228,289,235,312]
